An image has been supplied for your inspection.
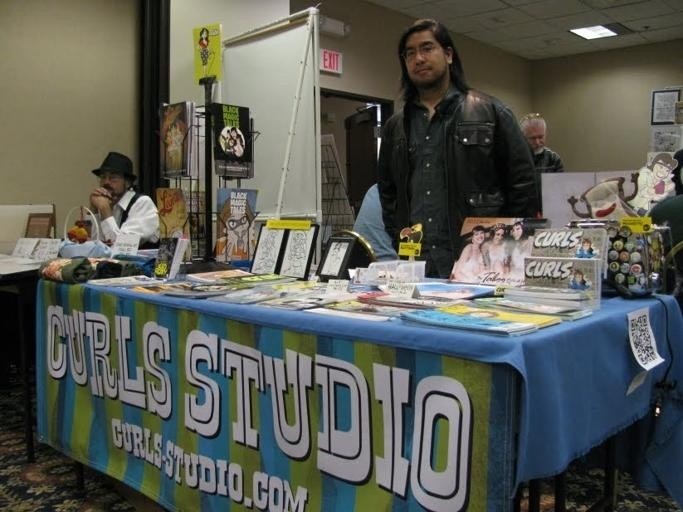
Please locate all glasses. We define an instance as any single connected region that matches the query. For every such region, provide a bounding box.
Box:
[520,112,544,123]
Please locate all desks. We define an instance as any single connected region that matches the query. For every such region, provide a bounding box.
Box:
[34,264,681,511]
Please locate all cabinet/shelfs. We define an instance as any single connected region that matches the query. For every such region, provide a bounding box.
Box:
[156,75,260,275]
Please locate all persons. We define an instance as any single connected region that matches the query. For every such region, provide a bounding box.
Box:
[376,19,539,279]
[648,194,683,297]
[575,240,597,258]
[521,112,563,170]
[352,184,399,262]
[569,270,591,291]
[84,152,160,250]
[89,268,592,337]
[451,221,535,282]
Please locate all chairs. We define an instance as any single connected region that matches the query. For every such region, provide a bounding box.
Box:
[323,231,377,268]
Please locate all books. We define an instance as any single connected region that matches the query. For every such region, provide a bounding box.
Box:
[210,101,259,261]
[155,100,207,263]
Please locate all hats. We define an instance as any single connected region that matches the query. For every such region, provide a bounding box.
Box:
[91,151,137,181]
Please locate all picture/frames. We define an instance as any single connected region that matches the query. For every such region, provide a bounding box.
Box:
[25,212,55,238]
[316,236,357,280]
[249,224,320,279]
[651,90,681,125]
